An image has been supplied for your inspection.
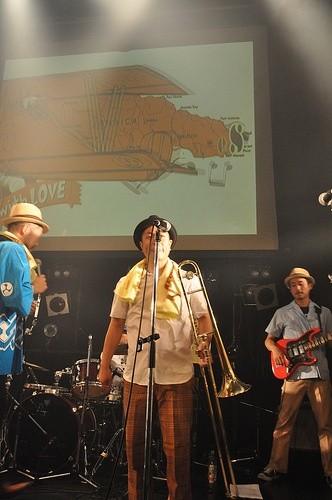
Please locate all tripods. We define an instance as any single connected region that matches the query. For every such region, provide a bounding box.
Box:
[0,372,170,491]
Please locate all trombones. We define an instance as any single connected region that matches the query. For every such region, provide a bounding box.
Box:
[175,259,255,499]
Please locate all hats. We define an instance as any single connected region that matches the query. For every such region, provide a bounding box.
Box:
[284,267,316,289]
[0,202,49,234]
[133,215,178,250]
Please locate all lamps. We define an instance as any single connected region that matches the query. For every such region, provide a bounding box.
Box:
[235,282,280,314]
[44,289,72,317]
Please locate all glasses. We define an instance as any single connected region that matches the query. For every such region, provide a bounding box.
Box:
[289,282,307,288]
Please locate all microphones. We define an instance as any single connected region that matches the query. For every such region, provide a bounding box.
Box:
[319,189,332,206]
[35,259,42,277]
[154,219,172,232]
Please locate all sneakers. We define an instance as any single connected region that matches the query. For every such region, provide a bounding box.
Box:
[324,474,332,488]
[256,466,282,481]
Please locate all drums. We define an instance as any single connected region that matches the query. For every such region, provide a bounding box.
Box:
[4,392,98,481]
[20,384,69,402]
[71,359,113,398]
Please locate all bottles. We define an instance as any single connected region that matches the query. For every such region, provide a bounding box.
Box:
[208,450,218,484]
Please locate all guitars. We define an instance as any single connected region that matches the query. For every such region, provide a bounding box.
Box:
[271,327,332,380]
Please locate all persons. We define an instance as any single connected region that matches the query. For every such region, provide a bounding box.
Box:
[99,215,212,500]
[0,202,50,457]
[255,267,332,489]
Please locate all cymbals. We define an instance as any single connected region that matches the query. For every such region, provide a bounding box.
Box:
[23,360,49,371]
